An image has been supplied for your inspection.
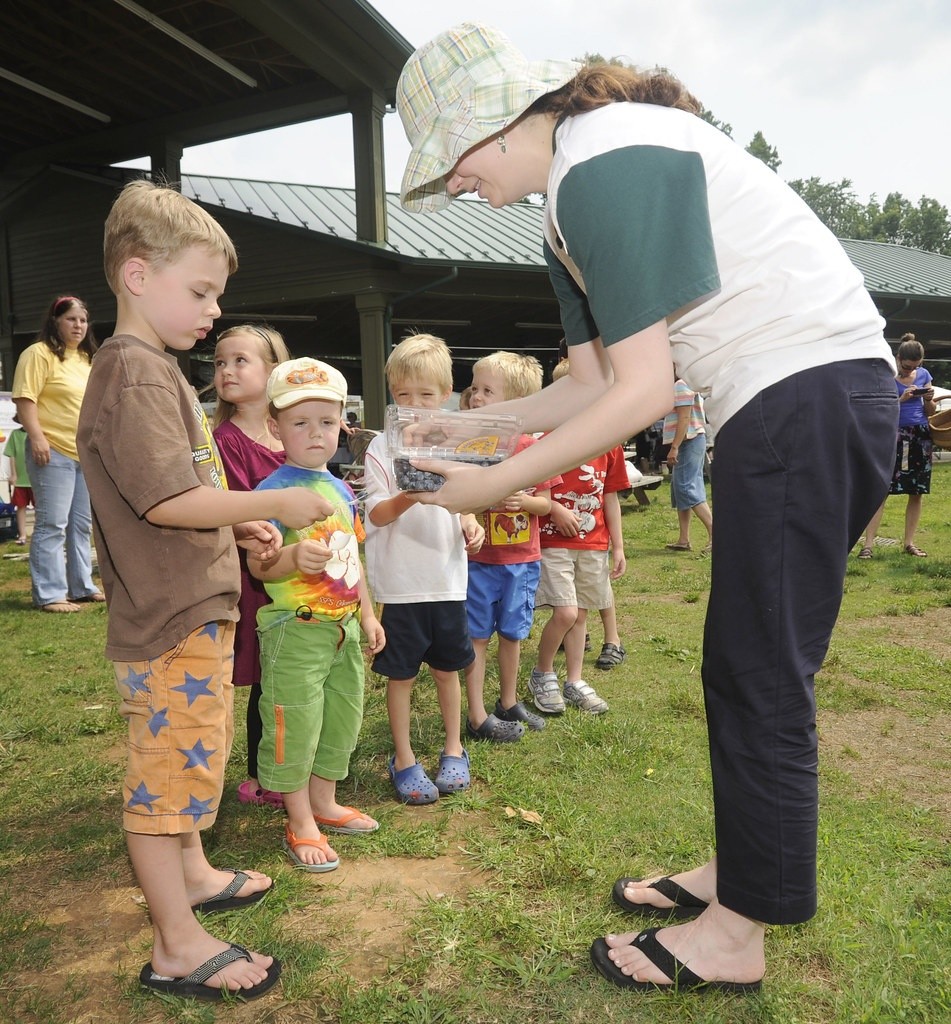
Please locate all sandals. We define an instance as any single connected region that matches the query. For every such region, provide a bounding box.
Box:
[434,747,470,792]
[312,805,379,833]
[464,713,525,744]
[492,698,545,731]
[282,823,342,871]
[38,602,81,611]
[389,753,439,804]
[77,591,106,602]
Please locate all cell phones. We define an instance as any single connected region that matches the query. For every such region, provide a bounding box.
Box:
[913,388,930,395]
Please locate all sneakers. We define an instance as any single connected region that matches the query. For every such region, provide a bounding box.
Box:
[595,643,626,669]
[558,633,591,652]
[563,680,610,715]
[527,666,565,715]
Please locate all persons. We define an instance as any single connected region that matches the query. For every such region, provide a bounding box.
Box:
[858,333,936,560]
[365,333,470,805]
[3,413,34,545]
[458,337,712,743]
[396,19,901,994]
[248,357,384,872]
[347,412,361,429]
[11,296,106,614]
[77,179,333,1003]
[211,324,290,809]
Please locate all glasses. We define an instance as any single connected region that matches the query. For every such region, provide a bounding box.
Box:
[899,358,924,371]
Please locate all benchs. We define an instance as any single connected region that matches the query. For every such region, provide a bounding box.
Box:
[620,475,664,497]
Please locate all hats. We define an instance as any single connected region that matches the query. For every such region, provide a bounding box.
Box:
[266,356,349,419]
[396,21,584,215]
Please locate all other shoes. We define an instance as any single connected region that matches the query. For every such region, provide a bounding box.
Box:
[700,543,713,553]
[665,542,692,551]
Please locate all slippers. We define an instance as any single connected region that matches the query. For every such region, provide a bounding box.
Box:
[590,928,761,993]
[614,877,709,917]
[857,548,872,560]
[14,538,26,545]
[902,545,928,557]
[238,782,286,808]
[190,868,278,910]
[140,941,282,1000]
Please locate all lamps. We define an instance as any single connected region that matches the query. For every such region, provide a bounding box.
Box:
[514,321,564,330]
[391,318,471,328]
[112,0,258,88]
[214,308,318,322]
[885,337,902,344]
[927,339,951,346]
[0,67,112,125]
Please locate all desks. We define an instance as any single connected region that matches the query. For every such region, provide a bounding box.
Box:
[623,451,648,506]
